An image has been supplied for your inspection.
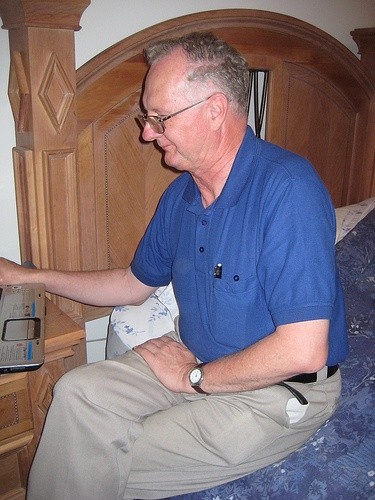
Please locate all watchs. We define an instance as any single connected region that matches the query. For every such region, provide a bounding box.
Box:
[189,362,212,396]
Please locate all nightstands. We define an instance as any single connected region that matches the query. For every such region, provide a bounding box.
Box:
[0,290,83,500]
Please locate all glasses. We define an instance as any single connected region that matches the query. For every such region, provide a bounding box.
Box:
[138,92,231,134]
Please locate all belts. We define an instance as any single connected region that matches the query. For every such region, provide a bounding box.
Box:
[278,364,339,407]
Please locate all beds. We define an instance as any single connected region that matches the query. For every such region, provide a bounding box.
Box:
[0,0,375,500]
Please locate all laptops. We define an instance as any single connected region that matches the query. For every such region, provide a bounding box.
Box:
[0,282,46,373]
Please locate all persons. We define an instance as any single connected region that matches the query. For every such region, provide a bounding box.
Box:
[0,31,350,500]
